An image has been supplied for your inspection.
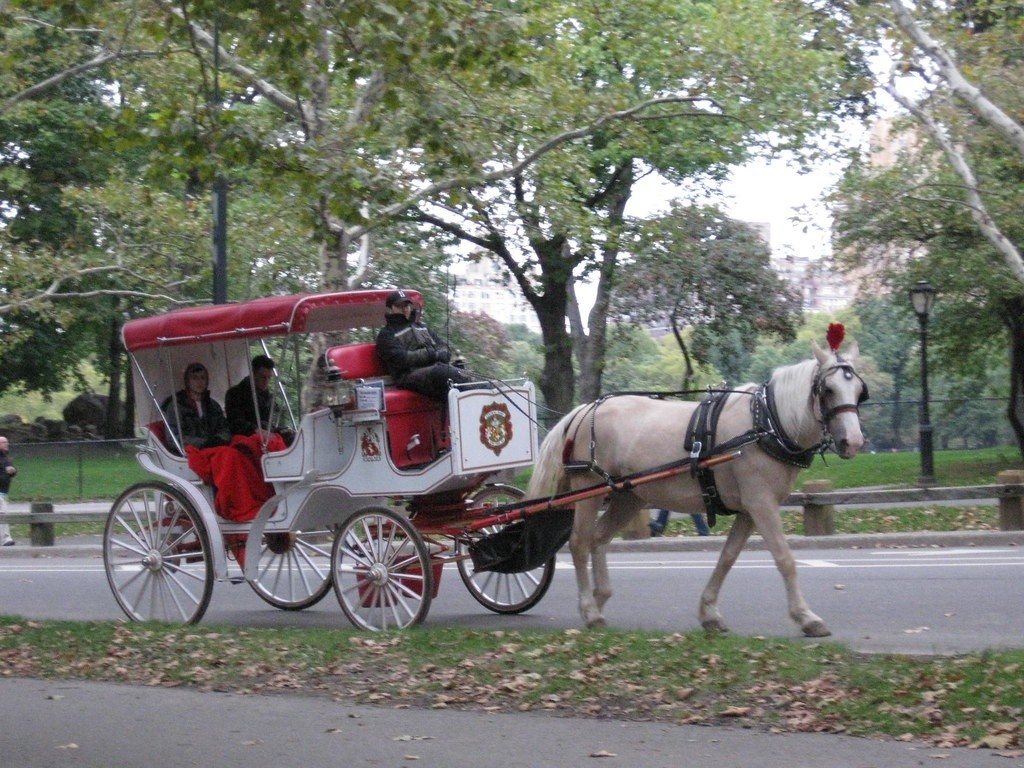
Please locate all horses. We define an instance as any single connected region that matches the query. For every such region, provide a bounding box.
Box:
[523,338,867,639]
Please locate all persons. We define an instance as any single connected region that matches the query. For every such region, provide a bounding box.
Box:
[0,436,17,545]
[224,355,296,446]
[651,509,710,536]
[162,363,287,524]
[375,290,493,404]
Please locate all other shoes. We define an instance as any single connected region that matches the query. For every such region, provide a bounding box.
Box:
[2,540,16,547]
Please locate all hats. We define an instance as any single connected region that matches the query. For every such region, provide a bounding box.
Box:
[386,290,413,306]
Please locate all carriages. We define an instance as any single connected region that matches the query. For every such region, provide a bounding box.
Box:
[103,288,869,635]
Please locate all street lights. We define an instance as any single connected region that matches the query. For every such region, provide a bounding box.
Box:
[909,280,939,484]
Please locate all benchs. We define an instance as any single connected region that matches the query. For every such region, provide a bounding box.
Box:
[137,419,215,485]
[317,343,448,417]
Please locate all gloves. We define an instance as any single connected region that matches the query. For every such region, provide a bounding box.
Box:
[433,350,452,363]
[203,436,226,447]
